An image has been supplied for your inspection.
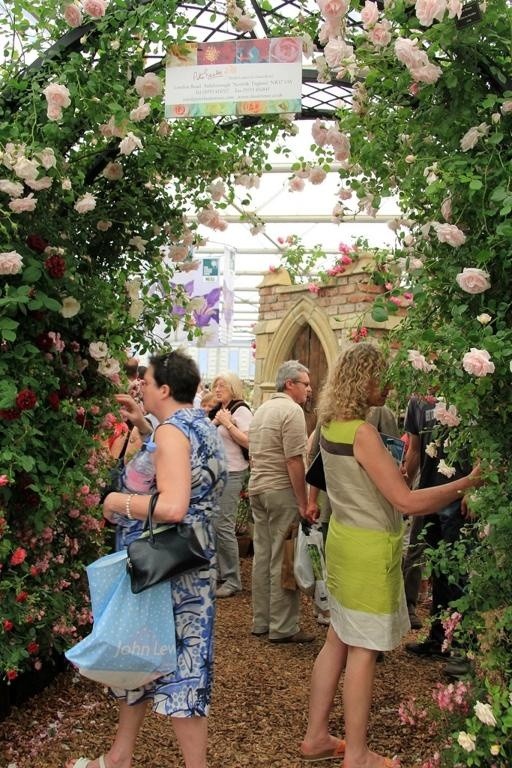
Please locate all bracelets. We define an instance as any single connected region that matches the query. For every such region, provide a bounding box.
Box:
[126,493,138,521]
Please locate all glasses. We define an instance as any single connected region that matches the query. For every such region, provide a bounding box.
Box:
[291,378,309,386]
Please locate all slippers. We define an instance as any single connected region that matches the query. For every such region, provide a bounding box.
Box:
[301,741,346,760]
[342,757,401,768]
[65,756,105,768]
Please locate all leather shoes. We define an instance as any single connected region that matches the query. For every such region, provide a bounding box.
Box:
[407,639,451,656]
[445,660,470,677]
[410,613,422,628]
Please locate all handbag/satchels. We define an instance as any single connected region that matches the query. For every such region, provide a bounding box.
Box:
[125,491,211,595]
[283,520,298,592]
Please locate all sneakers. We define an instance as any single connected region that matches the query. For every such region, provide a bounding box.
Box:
[252,629,269,636]
[312,603,319,617]
[216,582,240,597]
[318,613,331,624]
[270,628,315,642]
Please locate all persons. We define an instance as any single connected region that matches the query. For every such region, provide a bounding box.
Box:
[307,384,476,660]
[247,360,316,645]
[63,351,228,768]
[101,358,218,466]
[296,343,482,767]
[210,371,253,598]
